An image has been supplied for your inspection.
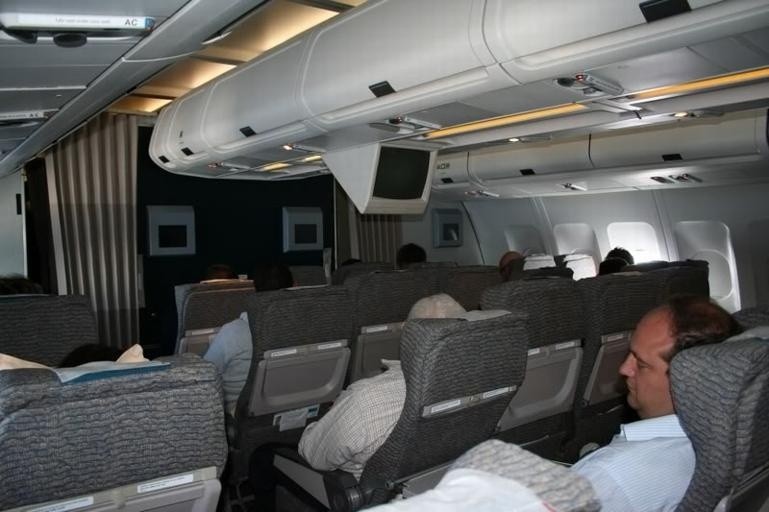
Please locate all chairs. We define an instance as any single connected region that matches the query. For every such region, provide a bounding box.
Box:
[0,289,110,367]
[175,258,769,512]
[0,356,229,512]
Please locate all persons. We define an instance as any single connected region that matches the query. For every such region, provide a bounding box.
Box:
[397,243,425,267]
[490,252,523,283]
[606,247,634,265]
[596,256,630,277]
[161,264,235,348]
[248,292,468,510]
[1,272,43,297]
[339,258,363,268]
[368,291,746,511]
[61,343,125,371]
[202,263,299,424]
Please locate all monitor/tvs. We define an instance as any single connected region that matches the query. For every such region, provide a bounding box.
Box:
[433,208,463,249]
[145,205,196,257]
[320,141,439,215]
[282,206,324,253]
